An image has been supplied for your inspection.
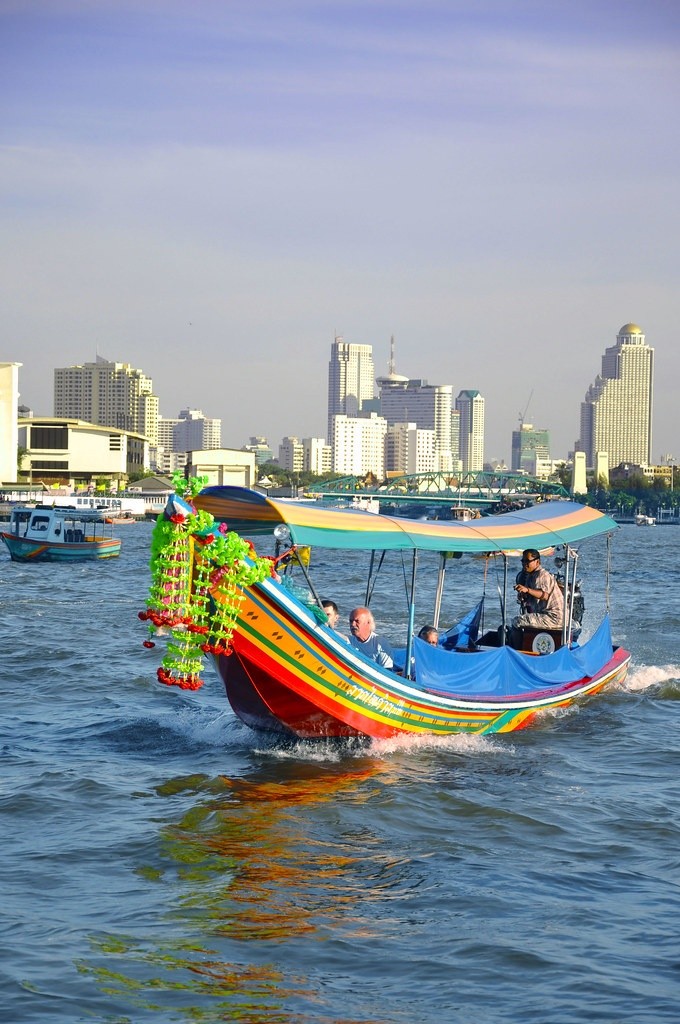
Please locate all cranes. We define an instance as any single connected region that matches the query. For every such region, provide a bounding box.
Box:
[517,388,536,424]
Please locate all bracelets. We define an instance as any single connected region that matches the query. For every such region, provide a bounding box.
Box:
[527,588,529,592]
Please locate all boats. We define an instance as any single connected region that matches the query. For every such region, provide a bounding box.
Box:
[145,505,164,522]
[0,505,122,563]
[450,506,482,521]
[152,484,637,743]
[11,507,120,522]
[634,515,656,527]
[106,517,135,524]
[483,547,556,558]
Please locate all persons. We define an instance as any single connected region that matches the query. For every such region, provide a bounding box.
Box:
[349,607,393,672]
[317,600,350,643]
[510,549,570,650]
[402,626,437,679]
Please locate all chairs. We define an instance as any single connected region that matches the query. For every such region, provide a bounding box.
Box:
[521,583,574,657]
[25,525,85,543]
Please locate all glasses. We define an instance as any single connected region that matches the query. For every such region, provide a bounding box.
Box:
[520,558,537,564]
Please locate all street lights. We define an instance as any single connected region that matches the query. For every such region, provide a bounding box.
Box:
[669,458,676,491]
